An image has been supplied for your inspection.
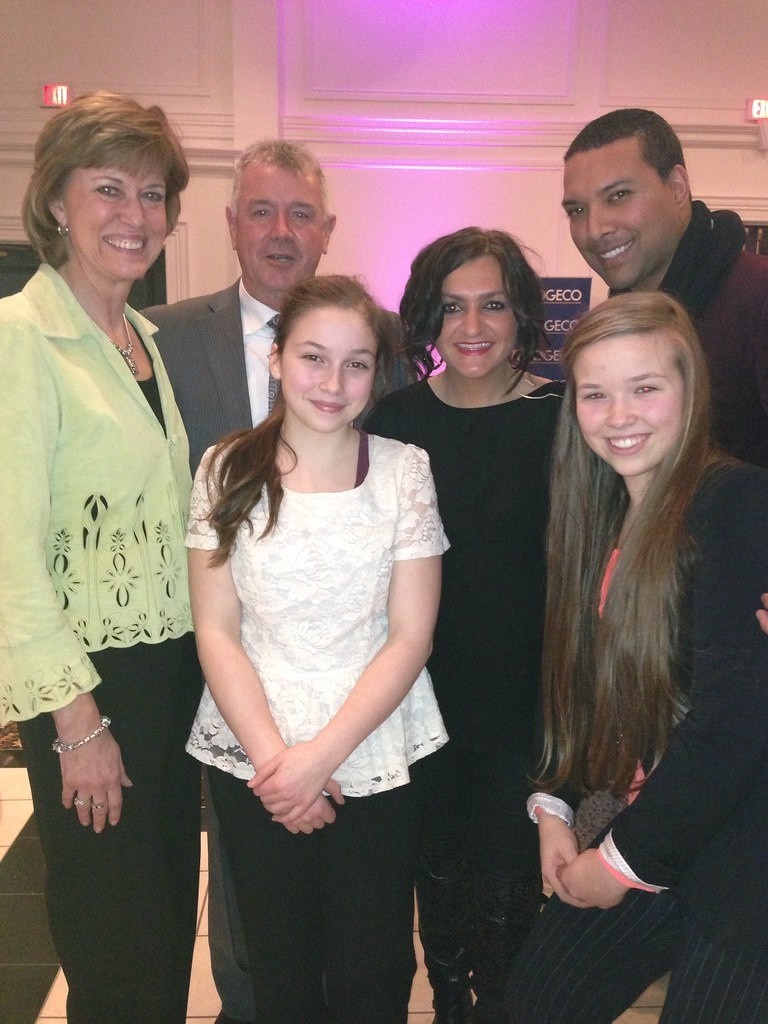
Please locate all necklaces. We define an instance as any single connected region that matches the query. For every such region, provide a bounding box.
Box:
[109,311,141,376]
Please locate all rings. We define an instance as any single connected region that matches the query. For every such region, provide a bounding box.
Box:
[72,797,92,808]
[90,801,108,810]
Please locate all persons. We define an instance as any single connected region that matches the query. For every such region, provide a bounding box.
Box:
[134,138,419,1023]
[480,290,768,1024]
[560,106,768,490]
[0,87,205,1024]
[359,226,586,1024]
[184,271,455,1024]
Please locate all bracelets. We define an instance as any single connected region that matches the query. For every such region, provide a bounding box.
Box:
[51,712,110,752]
